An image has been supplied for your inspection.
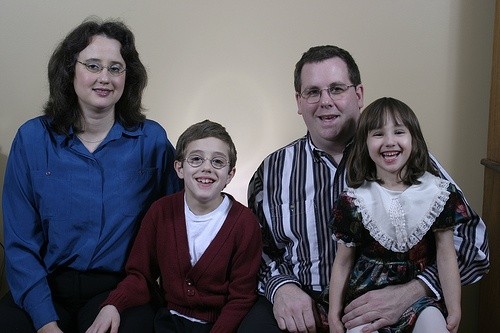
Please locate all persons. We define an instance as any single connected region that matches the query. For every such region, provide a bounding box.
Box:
[0,15,184,333]
[327,97,462,333]
[85,120,261,333]
[236,43,489,333]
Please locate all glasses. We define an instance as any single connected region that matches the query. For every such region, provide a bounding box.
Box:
[182,154,232,170]
[298,84,356,103]
[75,57,128,77]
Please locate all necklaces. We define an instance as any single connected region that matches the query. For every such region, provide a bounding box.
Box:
[76,134,105,143]
